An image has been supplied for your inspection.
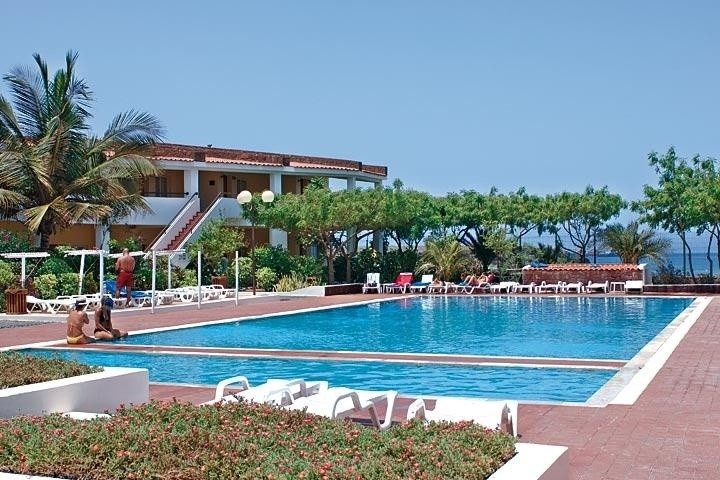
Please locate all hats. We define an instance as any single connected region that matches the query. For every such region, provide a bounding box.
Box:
[72,298,88,306]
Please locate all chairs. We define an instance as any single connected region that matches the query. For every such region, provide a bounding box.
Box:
[200,374,329,408]
[26,284,236,315]
[276,386,399,431]
[406,396,520,439]
[361,271,644,295]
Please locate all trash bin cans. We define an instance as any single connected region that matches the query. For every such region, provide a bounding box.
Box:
[5,289,28,315]
[211,276,228,289]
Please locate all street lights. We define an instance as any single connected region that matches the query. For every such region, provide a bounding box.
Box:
[236,189,273,296]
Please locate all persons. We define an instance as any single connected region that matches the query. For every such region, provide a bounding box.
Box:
[94,297,128,339]
[432,277,442,284]
[115,247,136,309]
[67,297,96,345]
[459,274,495,286]
